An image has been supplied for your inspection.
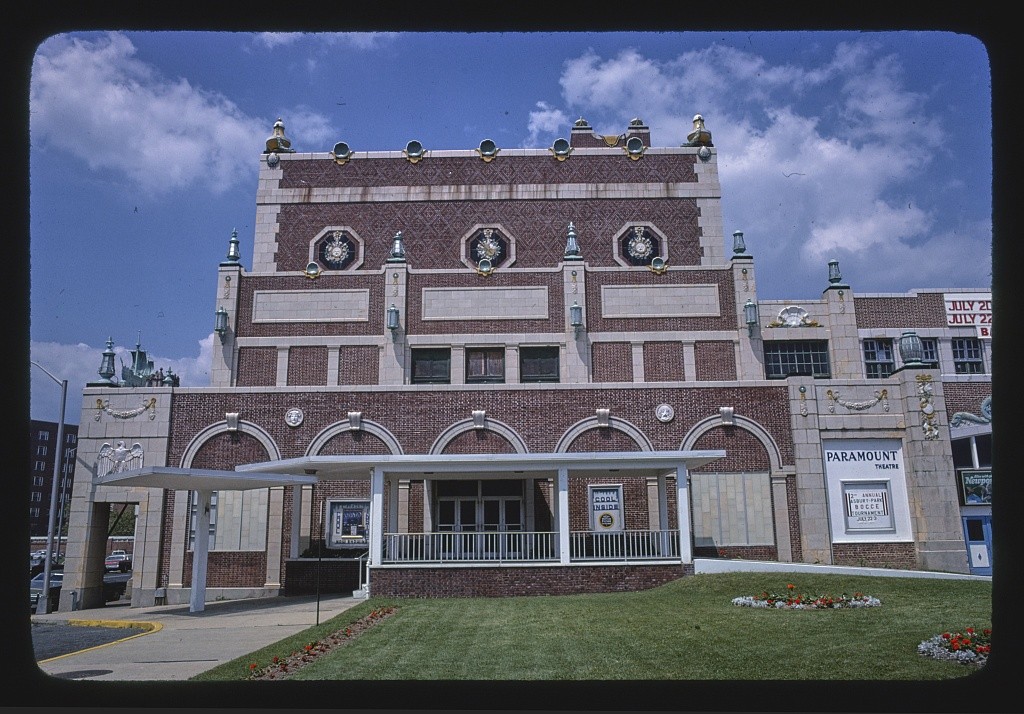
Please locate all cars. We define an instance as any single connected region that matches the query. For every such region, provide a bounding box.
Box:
[30,569,127,607]
[31,550,63,562]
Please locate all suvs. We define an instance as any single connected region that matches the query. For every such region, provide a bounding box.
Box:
[106,555,133,574]
